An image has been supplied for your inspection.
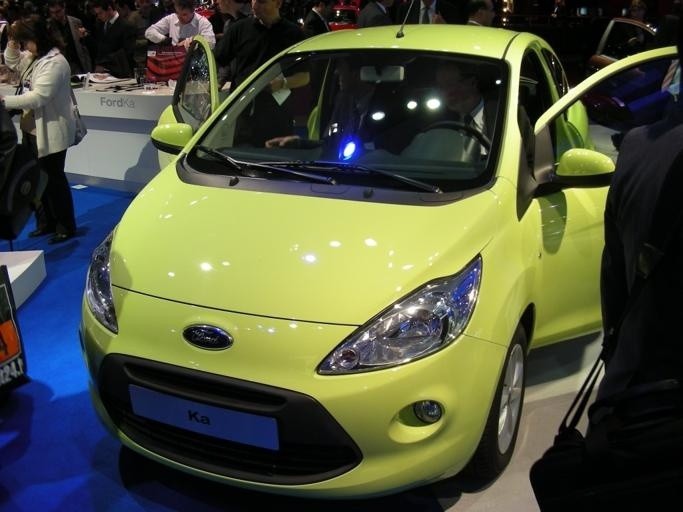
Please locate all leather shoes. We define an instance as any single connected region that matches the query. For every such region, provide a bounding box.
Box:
[28,227,78,245]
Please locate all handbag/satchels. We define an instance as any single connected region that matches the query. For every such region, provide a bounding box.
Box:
[145,45,192,82]
[528,344,614,512]
[70,86,88,146]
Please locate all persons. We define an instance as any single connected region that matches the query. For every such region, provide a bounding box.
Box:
[357,1,520,30]
[437,64,537,177]
[42,2,91,77]
[264,51,401,153]
[303,1,335,38]
[586,20,683,512]
[87,2,248,90]
[1,15,86,242]
[218,1,312,147]
[615,2,650,59]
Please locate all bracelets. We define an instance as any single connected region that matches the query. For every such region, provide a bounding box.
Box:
[283,78,287,88]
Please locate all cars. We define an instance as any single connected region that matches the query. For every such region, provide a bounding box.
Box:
[78,5,683,501]
[312,0,683,131]
